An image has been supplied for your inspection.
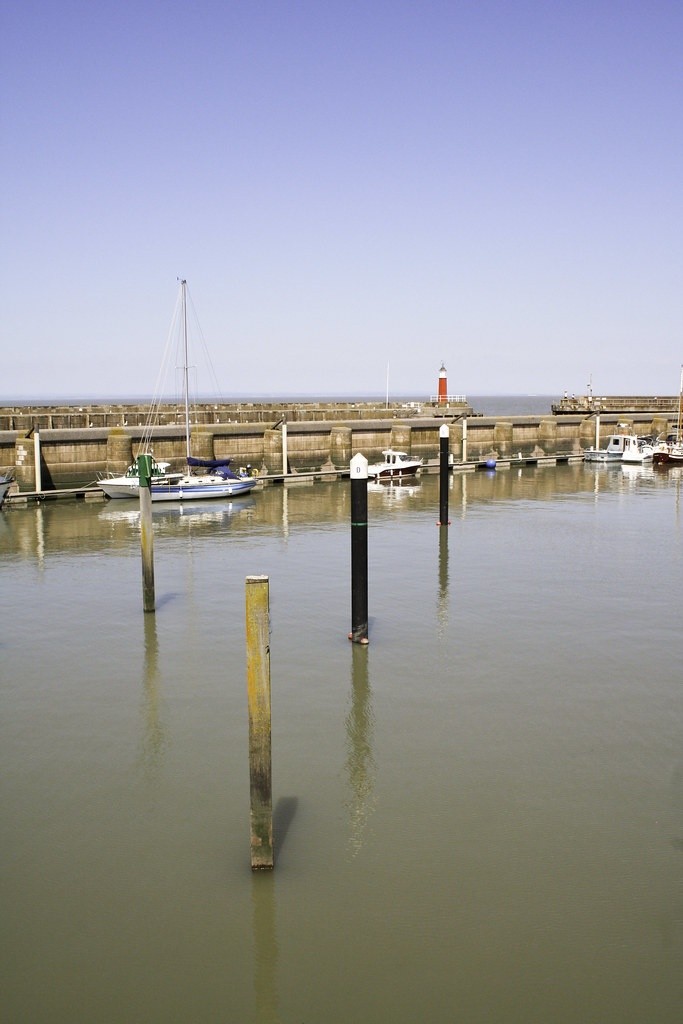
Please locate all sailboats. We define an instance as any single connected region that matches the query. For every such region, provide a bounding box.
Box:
[124,277,260,501]
[653,363,683,464]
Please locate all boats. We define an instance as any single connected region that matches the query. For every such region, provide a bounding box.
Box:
[620,434,655,462]
[369,450,424,480]
[94,451,184,499]
[0,465,15,509]
[583,423,655,461]
